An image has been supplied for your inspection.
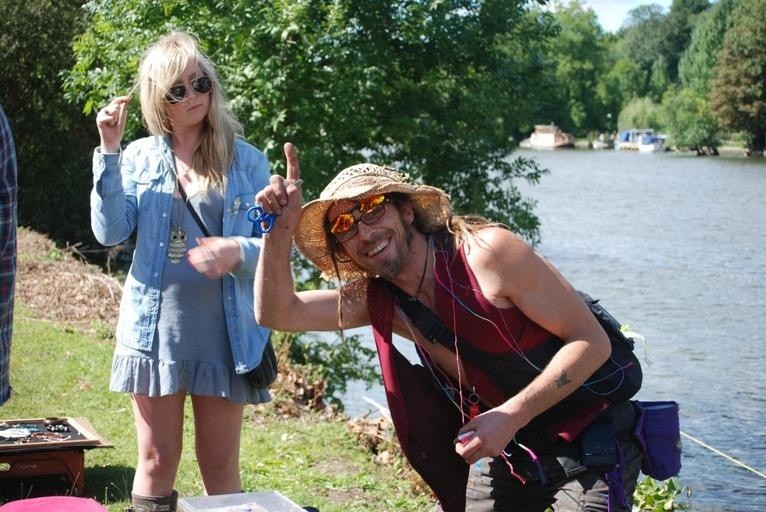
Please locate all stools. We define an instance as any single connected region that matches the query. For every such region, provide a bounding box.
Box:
[0,449,84,499]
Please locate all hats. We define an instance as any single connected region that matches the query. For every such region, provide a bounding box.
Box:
[293,163,451,282]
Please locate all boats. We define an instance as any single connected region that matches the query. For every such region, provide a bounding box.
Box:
[515,119,671,154]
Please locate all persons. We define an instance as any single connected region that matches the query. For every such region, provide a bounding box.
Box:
[89,33,276,512]
[252,142,644,511]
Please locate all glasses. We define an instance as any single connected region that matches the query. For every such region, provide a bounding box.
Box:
[165,76,211,104]
[330,194,390,243]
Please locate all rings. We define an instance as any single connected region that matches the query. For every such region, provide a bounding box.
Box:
[104,108,109,115]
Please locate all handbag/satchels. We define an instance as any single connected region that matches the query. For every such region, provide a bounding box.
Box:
[245,339,277,390]
[485,290,642,400]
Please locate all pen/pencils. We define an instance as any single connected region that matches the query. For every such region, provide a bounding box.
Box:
[453,430,476,444]
[195,237,237,280]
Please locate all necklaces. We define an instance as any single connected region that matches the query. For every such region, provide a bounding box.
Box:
[166,152,197,265]
[405,236,429,306]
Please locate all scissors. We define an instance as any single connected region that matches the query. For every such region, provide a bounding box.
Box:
[247,179,303,233]
